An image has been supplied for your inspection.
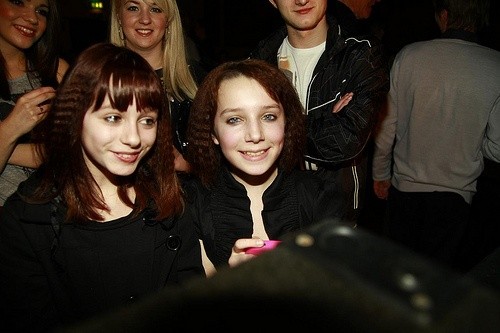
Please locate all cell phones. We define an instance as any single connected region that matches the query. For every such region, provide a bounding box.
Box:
[246,240,283,256]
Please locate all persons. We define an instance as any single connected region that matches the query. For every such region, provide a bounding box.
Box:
[110,0,198,177]
[181,58,350,269]
[0,43,232,333]
[0,0,71,205]
[246,0,390,229]
[370,0,500,273]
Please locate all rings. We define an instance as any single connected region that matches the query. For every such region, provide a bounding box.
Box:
[39,105,44,113]
